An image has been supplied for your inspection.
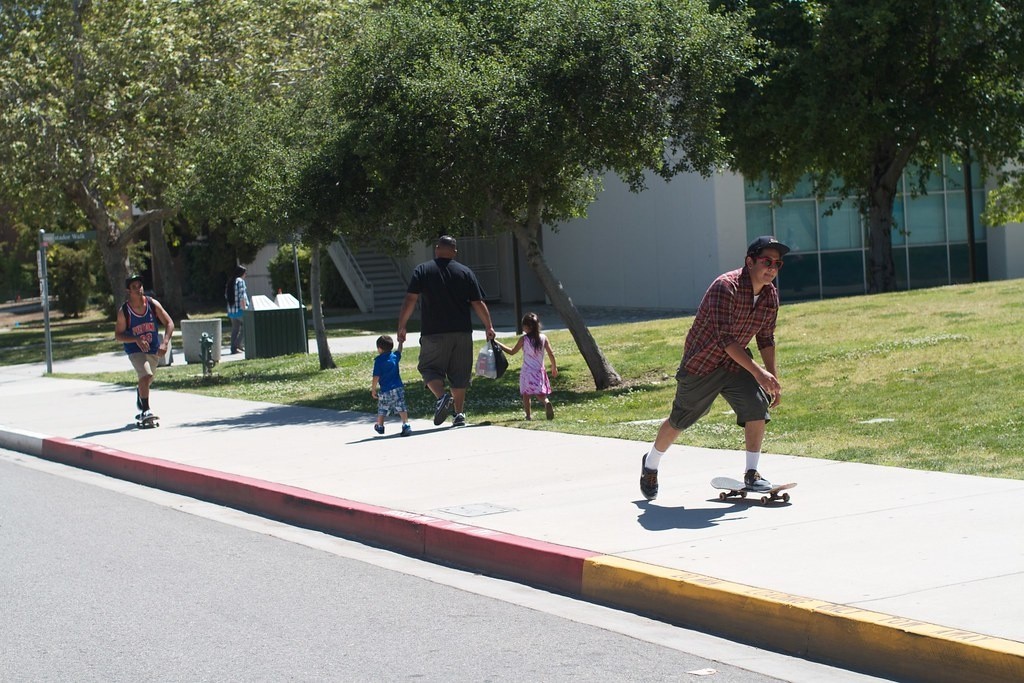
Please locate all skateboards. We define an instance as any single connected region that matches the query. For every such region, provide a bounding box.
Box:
[709,476,798,506]
[135,413,160,429]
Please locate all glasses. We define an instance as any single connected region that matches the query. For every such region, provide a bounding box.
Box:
[753,255,783,270]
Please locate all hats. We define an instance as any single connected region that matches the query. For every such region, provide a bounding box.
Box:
[747,237,790,256]
[126,274,143,288]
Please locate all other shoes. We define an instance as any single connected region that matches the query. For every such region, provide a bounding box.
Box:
[232,349,242,354]
[241,347,245,351]
[545,402,554,421]
[136,387,143,409]
[140,410,153,419]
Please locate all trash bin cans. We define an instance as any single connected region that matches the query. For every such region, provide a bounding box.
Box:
[243,293,310,360]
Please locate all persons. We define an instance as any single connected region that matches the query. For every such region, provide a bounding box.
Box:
[225,265,249,354]
[115,275,174,417]
[372,335,413,436]
[488,312,558,420]
[397,236,495,425]
[639,236,791,500]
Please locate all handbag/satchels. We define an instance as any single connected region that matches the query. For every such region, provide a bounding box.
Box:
[491,339,509,377]
[475,340,498,380]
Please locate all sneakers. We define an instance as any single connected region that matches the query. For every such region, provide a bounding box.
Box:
[374,424,385,434]
[744,469,772,493]
[400,424,413,437]
[448,413,467,429]
[639,452,658,501]
[434,393,450,425]
